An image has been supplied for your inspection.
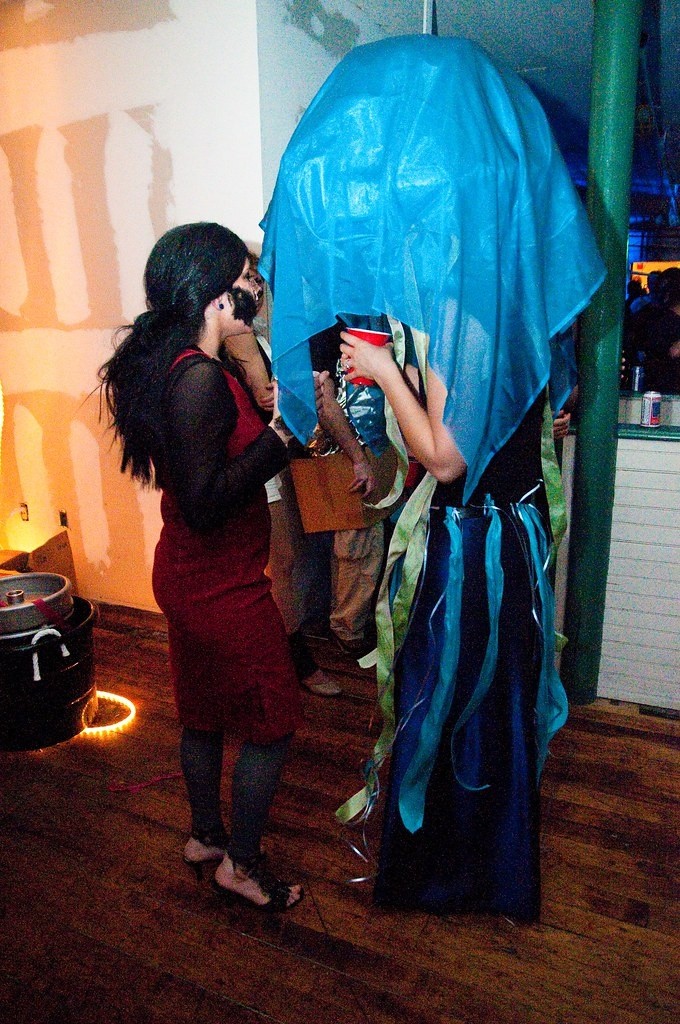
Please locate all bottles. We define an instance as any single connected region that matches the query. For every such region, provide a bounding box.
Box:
[628,351,646,392]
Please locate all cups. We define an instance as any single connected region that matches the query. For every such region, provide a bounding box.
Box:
[344,327,392,385]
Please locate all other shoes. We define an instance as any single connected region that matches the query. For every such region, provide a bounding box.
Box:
[301,669,344,696]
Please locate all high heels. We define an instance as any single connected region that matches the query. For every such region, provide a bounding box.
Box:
[181,834,228,880]
[211,850,304,912]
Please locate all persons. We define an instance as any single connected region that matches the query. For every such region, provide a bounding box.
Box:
[245,251,345,696]
[273,317,378,646]
[329,523,384,656]
[620,267,680,397]
[341,283,561,924]
[102,223,329,917]
[553,410,571,438]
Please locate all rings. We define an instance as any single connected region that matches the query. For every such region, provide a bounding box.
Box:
[345,360,351,370]
